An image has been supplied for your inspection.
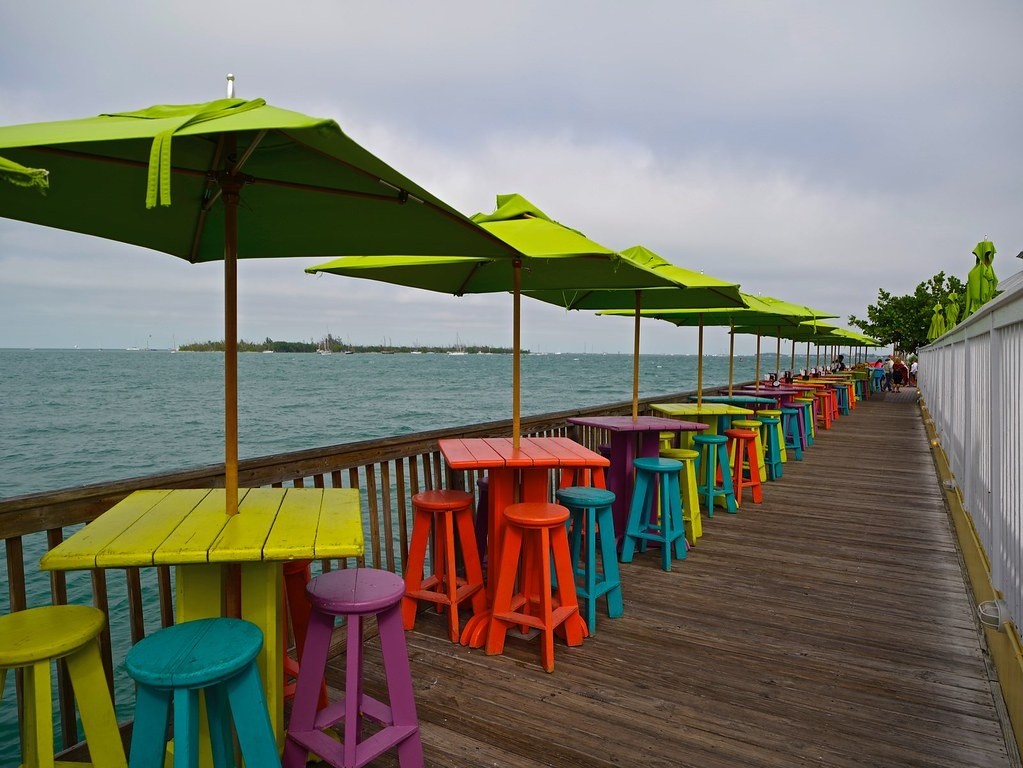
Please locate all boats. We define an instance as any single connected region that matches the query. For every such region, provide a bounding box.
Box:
[126,346,139,351]
[262,350,273,354]
[381,350,394,354]
[411,351,421,355]
[345,351,353,354]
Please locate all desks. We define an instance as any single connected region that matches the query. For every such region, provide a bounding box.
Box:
[436,436,610,651]
[825,374,852,380]
[761,382,826,397]
[648,403,755,510]
[835,370,866,401]
[793,379,836,428]
[743,385,814,408]
[793,376,845,386]
[688,395,778,436]
[38,488,366,768]
[566,416,710,554]
[718,389,797,418]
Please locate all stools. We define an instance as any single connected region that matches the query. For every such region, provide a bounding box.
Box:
[0,377,869,768]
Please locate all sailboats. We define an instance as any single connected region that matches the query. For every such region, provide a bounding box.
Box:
[316,336,332,356]
[446,331,469,356]
[170,333,180,353]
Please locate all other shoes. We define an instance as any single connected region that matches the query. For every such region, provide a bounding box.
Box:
[892,390,896,393]
[888,389,892,391]
[881,388,885,392]
[896,391,901,393]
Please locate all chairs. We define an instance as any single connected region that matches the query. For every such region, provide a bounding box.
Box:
[867,369,884,393]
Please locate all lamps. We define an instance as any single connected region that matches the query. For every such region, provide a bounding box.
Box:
[976,599,1011,633]
[930,438,940,449]
[942,478,956,493]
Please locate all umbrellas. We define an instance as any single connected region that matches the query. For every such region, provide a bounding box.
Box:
[959,278,976,320]
[678,291,888,407]
[968,235,999,313]
[943,289,961,331]
[0,74,530,768]
[510,245,751,482]
[306,193,686,596]
[596,289,842,422]
[926,301,946,342]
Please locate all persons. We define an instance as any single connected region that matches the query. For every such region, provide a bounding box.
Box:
[891,357,908,394]
[830,355,845,372]
[873,358,883,368]
[910,359,919,382]
[883,354,910,393]
